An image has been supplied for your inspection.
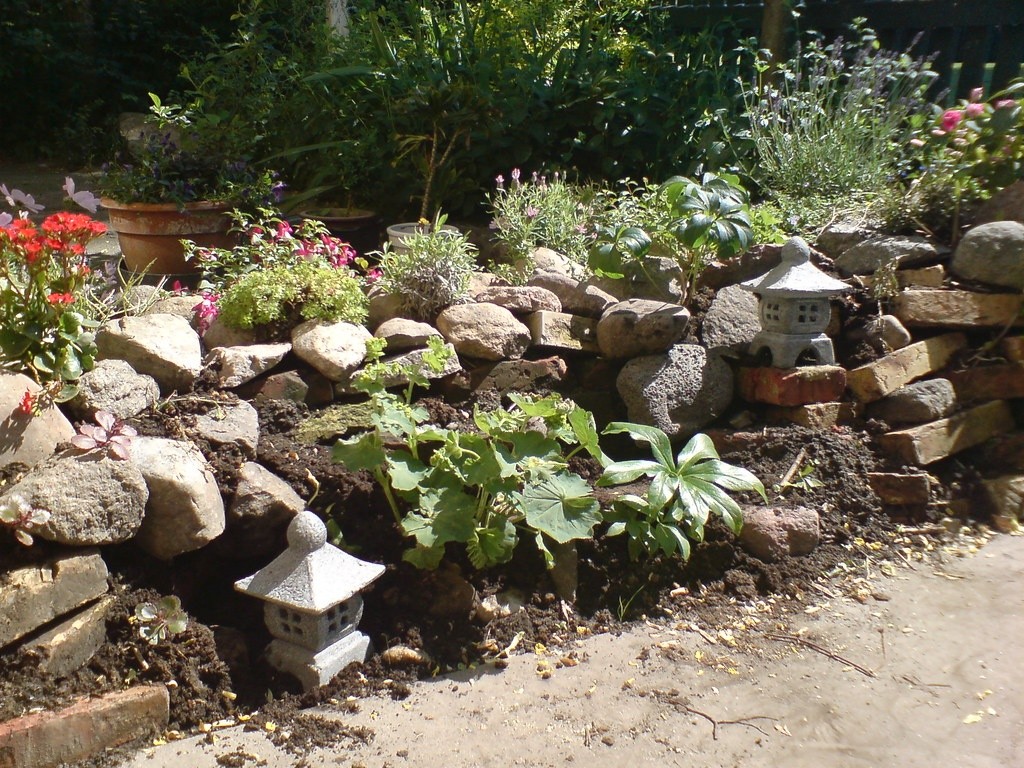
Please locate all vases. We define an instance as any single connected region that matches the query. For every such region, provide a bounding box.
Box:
[100,193,244,279]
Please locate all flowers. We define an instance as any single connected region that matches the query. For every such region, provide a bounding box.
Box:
[84,128,285,218]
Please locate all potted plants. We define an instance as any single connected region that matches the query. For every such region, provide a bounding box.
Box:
[296,133,389,254]
[386,11,522,258]
